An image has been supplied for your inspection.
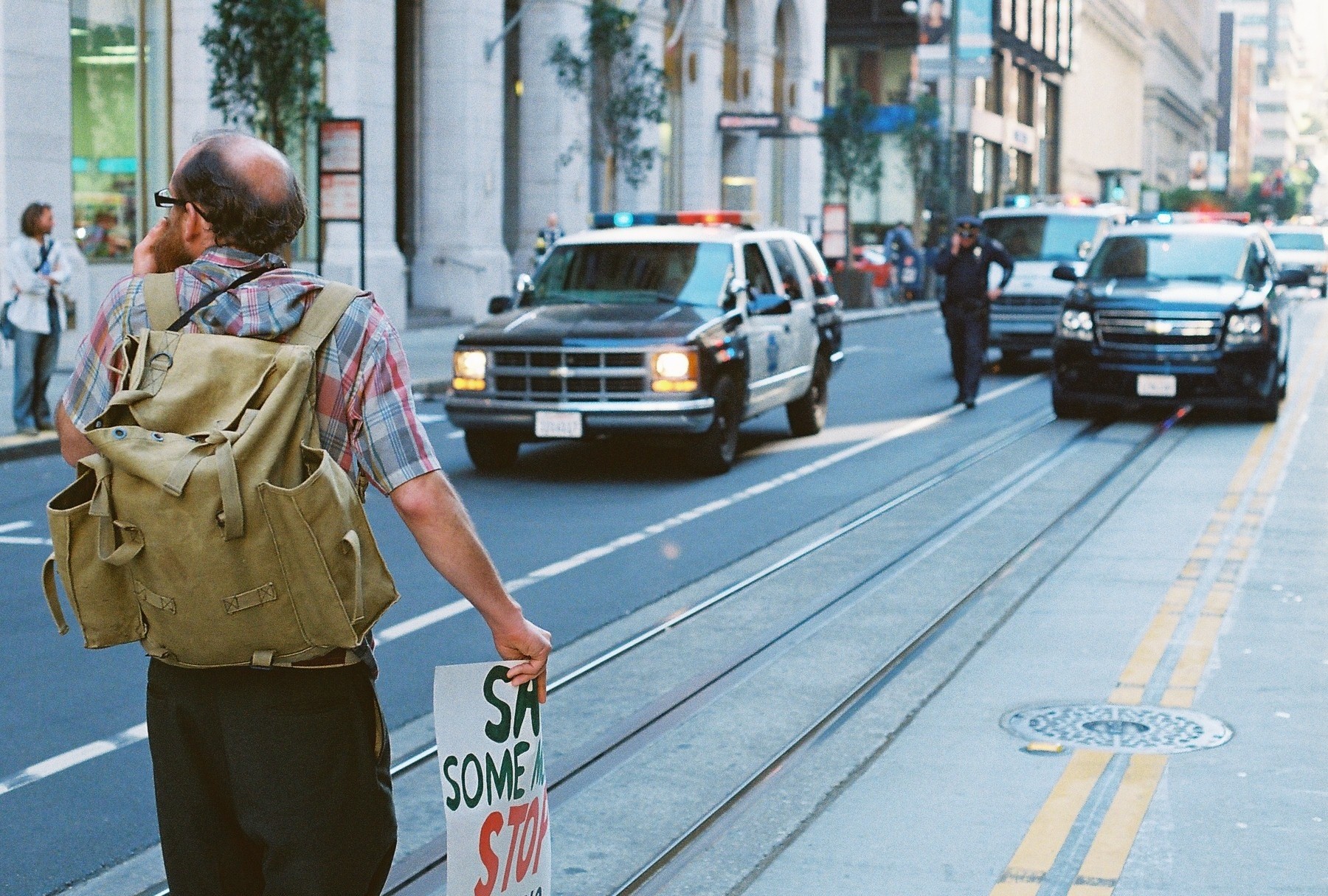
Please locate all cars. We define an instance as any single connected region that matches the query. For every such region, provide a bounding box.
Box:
[975,192,1328,375]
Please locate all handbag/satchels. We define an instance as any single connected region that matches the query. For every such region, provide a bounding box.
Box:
[0,301,16,340]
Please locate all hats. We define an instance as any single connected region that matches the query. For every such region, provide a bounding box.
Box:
[956,216,982,239]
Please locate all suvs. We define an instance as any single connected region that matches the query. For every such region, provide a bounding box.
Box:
[441,208,848,480]
[1051,207,1290,423]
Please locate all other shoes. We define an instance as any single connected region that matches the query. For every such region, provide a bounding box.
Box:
[964,396,975,408]
[954,391,965,404]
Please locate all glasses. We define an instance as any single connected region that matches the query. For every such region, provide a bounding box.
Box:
[154,188,211,224]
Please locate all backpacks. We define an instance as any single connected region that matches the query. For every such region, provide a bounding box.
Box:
[40,269,400,671]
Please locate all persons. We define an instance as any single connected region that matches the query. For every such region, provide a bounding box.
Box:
[6,202,74,435]
[883,222,913,262]
[56,133,553,896]
[78,210,131,259]
[918,0,951,46]
[932,216,1016,410]
